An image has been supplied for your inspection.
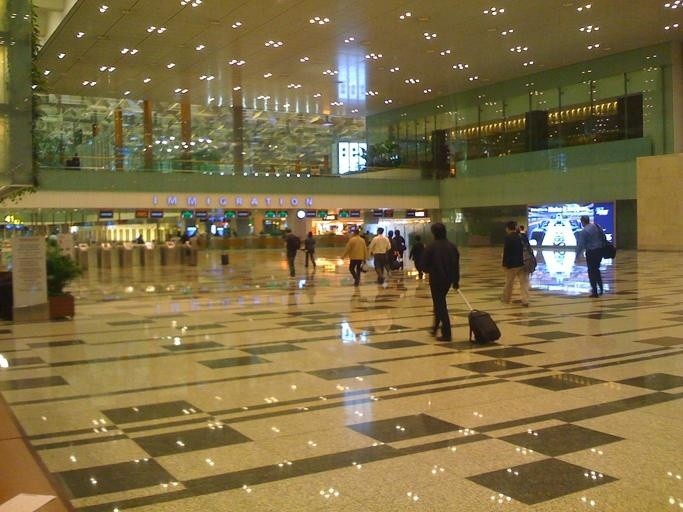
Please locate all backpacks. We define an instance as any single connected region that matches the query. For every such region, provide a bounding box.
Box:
[519,233,537,272]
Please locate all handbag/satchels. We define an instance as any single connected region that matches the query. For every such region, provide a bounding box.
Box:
[602,240,616,259]
[360,264,368,272]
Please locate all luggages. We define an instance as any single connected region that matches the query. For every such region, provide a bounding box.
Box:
[468,309,500,344]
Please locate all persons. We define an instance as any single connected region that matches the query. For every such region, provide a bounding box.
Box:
[519,224,526,238]
[368,228,425,285]
[356,225,363,233]
[223,220,231,235]
[574,215,607,298]
[498,220,531,307]
[137,234,145,244]
[340,230,368,287]
[283,228,299,277]
[304,231,316,268]
[180,236,193,248]
[72,152,80,170]
[420,221,461,342]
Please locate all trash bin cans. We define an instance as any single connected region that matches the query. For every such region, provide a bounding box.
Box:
[221,254,228,265]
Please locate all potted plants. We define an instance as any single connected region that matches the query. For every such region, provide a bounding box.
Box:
[370,139,401,166]
[46,237,84,320]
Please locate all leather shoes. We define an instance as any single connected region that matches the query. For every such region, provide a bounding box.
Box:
[430,331,450,341]
[590,294,597,296]
